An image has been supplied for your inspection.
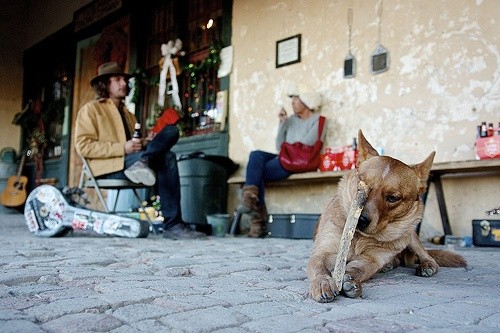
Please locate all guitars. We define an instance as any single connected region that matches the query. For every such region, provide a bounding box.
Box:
[0,149,28,207]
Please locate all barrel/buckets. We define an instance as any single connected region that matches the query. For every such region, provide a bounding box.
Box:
[207,213,231,237]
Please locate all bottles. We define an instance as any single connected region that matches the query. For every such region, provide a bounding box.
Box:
[132,123,142,138]
[476,122,500,140]
[427,235,465,245]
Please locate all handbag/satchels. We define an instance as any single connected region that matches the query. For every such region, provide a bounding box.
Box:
[280,116,324,172]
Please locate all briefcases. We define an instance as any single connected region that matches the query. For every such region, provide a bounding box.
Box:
[471,219,500,247]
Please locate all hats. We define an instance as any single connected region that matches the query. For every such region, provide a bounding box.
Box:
[90,61,135,87]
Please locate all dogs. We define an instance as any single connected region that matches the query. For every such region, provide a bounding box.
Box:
[308,130,468,303]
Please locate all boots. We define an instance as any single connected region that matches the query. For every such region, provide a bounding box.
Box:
[238,185,260,216]
[247,205,268,238]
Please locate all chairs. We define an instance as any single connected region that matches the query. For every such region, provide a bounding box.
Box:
[75,146,158,237]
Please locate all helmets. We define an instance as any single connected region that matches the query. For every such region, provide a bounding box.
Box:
[288,83,322,113]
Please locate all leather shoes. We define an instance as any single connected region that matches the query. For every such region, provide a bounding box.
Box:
[162,222,207,240]
[124,160,157,186]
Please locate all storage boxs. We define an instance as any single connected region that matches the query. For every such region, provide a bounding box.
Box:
[472,213,500,247]
[266,214,319,240]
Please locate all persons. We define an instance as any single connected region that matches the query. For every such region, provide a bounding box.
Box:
[74,61,207,240]
[233,81,329,237]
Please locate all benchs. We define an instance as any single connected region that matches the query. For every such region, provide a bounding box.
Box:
[226,156,500,240]
